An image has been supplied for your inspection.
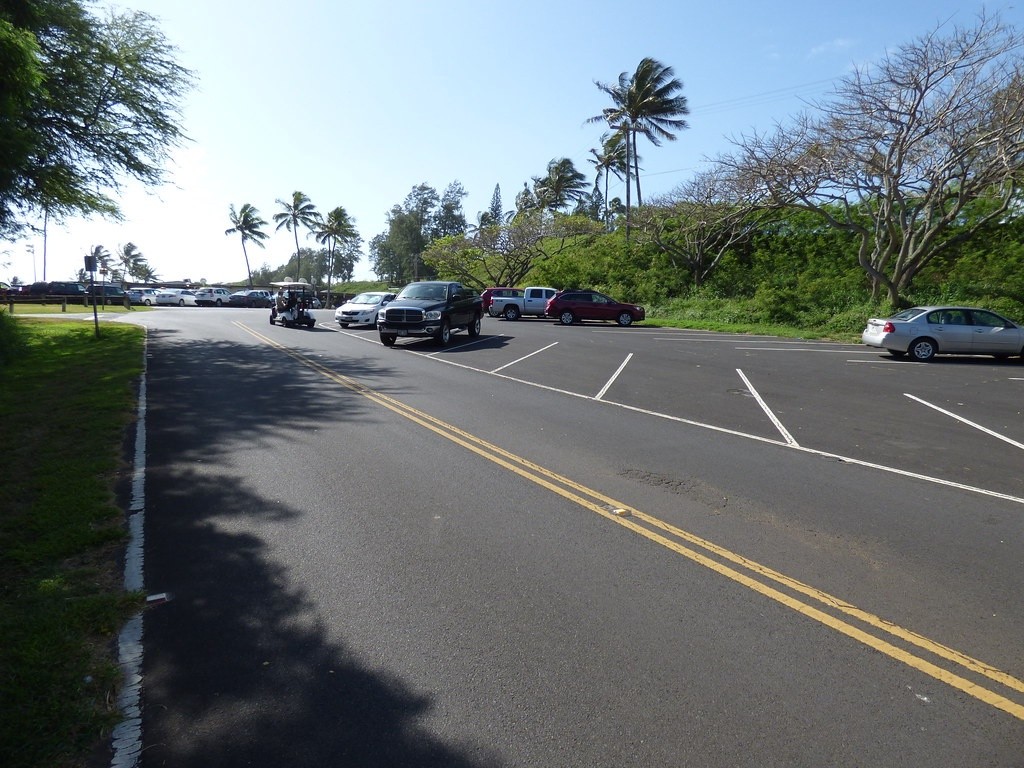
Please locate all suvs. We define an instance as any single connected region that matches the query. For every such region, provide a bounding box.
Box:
[545,287,645,327]
[194,286,233,308]
[229,289,271,309]
[480,286,527,319]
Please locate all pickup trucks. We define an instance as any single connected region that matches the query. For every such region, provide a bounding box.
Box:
[377,280,485,348]
[489,285,561,320]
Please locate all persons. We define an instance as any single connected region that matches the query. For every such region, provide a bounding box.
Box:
[275,292,286,308]
[288,293,297,307]
[946,313,953,320]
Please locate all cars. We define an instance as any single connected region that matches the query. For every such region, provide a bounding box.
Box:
[334,290,398,328]
[861,305,1024,362]
[306,296,323,310]
[0,278,198,307]
